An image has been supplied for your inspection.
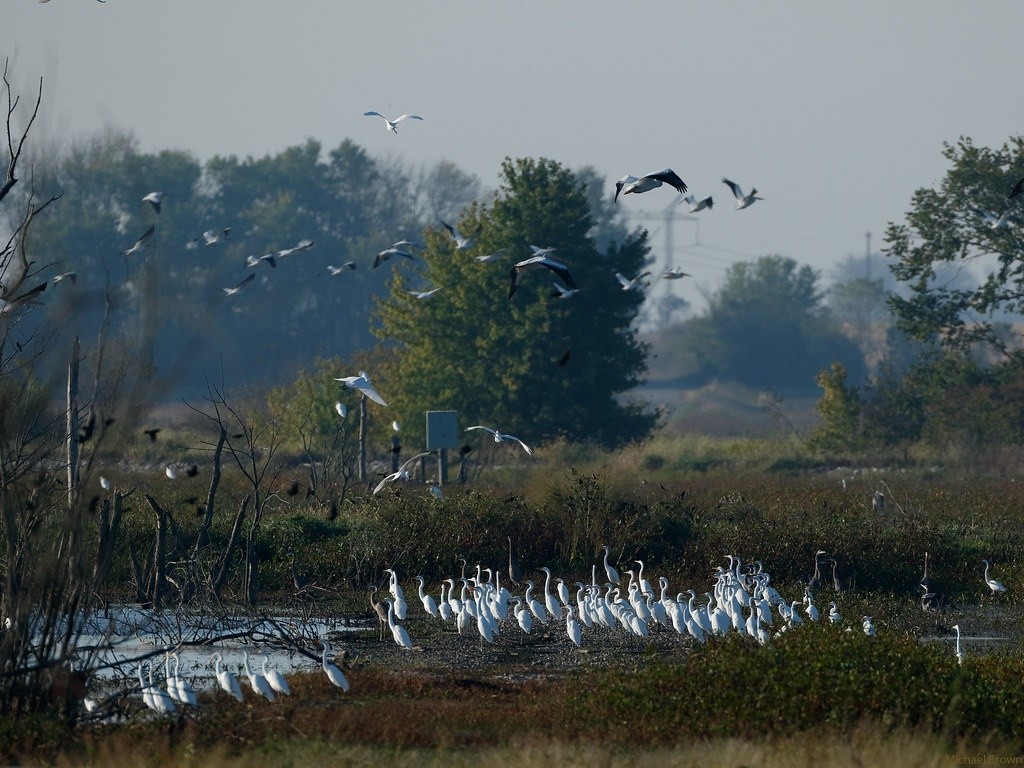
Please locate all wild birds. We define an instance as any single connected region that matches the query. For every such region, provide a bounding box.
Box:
[663,266,689,280]
[916,551,1006,665]
[372,450,430,497]
[614,270,652,292]
[334,373,386,406]
[614,168,688,203]
[331,399,347,418]
[364,112,423,134]
[406,282,445,300]
[438,220,483,251]
[365,536,875,654]
[123,189,418,296]
[721,179,764,211]
[679,194,714,213]
[51,271,77,288]
[135,648,290,714]
[464,425,532,455]
[319,641,350,693]
[476,245,579,303]
[90,416,338,522]
